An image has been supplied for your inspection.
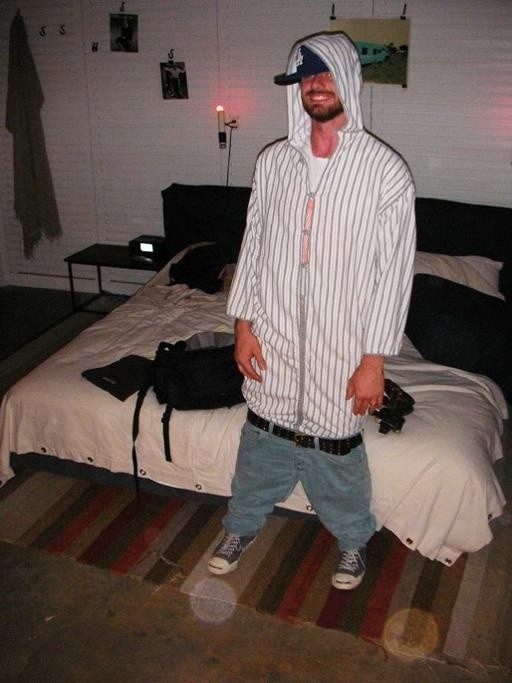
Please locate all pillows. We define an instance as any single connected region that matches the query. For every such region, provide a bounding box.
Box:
[415,250,504,300]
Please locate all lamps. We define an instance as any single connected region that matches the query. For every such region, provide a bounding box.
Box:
[217,105,227,151]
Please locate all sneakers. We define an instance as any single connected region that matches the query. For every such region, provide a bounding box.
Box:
[330,545,371,591]
[208,529,261,574]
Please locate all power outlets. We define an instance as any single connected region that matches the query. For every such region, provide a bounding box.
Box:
[230,116,239,129]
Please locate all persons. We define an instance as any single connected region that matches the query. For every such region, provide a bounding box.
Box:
[161,64,185,100]
[115,15,135,52]
[205,26,419,591]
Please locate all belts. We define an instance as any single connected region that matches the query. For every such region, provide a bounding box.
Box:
[243,405,363,456]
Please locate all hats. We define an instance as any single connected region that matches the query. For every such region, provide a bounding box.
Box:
[270,43,332,86]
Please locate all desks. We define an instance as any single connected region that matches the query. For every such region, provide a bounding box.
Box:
[63,244,161,314]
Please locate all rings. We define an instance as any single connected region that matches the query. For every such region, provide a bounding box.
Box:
[369,400,378,407]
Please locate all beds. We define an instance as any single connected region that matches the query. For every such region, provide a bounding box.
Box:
[0,184,512,567]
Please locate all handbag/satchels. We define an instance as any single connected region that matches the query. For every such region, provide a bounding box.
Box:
[78,352,155,403]
[149,328,252,413]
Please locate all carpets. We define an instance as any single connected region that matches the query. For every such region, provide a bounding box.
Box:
[0,293,512,678]
